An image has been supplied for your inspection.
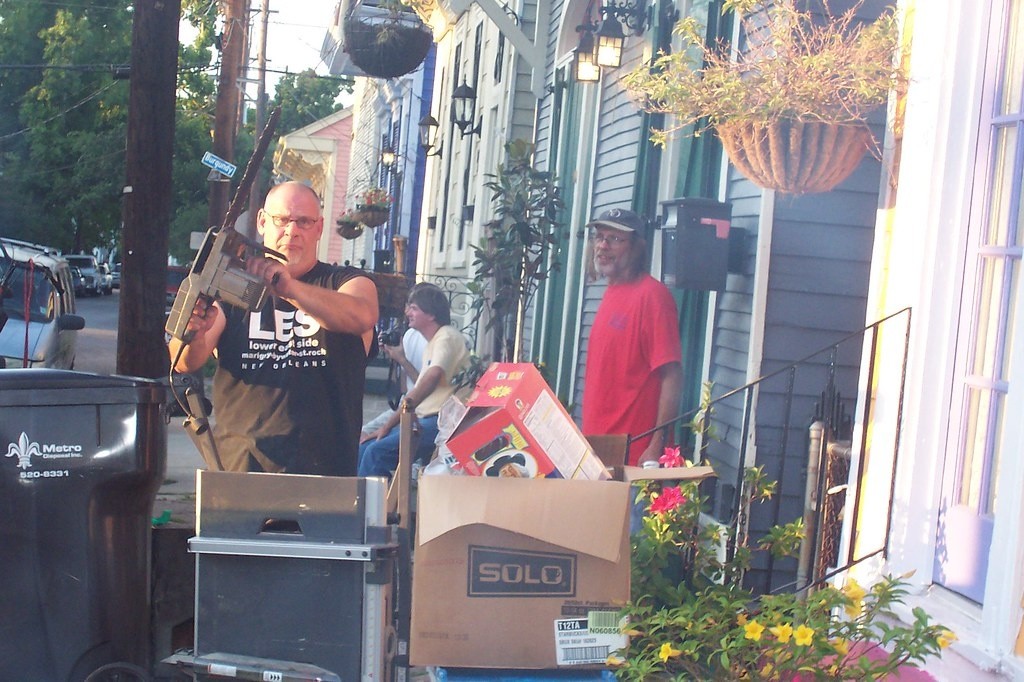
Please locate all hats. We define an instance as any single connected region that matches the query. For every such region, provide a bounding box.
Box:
[585,209,646,238]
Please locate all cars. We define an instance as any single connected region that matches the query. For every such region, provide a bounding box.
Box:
[166,267,190,304]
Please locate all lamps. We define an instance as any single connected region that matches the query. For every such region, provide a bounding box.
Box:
[382,142,403,181]
[451,77,482,140]
[572,8,601,82]
[418,113,443,159]
[595,0,653,67]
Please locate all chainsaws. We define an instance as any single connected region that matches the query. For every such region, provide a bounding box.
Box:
[165,104,291,346]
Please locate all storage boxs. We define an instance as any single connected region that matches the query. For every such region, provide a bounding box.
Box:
[443,360,615,485]
[411,431,714,670]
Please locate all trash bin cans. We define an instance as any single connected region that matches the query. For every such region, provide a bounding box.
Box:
[0,366,206,682]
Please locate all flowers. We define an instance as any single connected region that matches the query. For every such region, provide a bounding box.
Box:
[353,187,396,209]
[336,209,362,223]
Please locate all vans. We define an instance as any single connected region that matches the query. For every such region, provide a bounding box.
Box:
[0,239,86,370]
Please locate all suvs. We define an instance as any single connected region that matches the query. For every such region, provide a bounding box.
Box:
[61,255,122,298]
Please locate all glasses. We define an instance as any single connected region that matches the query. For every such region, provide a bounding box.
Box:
[593,233,632,243]
[264,210,319,229]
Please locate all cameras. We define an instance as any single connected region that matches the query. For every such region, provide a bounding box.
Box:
[379,328,401,346]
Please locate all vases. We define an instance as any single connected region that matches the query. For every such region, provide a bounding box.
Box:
[335,221,363,239]
[358,206,389,228]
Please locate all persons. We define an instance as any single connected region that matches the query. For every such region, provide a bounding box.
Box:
[358,280,472,476]
[167,180,379,477]
[580,210,683,540]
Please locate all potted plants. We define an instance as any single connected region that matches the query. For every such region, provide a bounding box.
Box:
[615,0,916,193]
